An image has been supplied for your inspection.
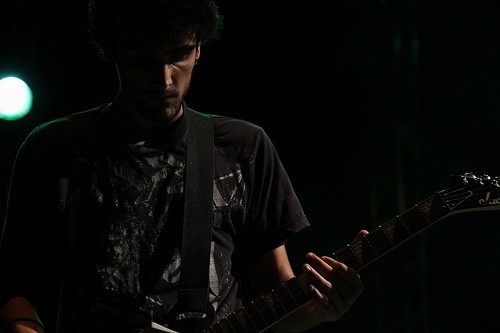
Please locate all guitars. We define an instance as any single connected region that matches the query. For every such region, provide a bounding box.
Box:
[197,170,500,333]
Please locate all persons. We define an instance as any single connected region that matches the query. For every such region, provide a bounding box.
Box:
[0,1,370,333]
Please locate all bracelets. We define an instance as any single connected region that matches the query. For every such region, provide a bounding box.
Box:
[6,317,45,332]
